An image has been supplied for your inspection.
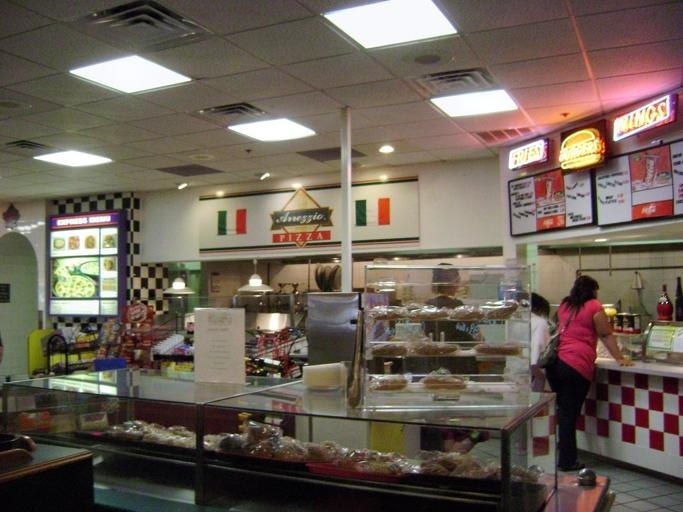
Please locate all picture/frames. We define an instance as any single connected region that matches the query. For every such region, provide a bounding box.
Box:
[0,443,95,512]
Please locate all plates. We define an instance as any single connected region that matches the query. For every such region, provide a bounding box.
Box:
[0,366,614,512]
[364,262,532,466]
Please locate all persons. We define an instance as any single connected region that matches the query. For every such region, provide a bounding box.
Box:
[546,275,634,472]
[425,263,486,373]
[530,292,549,393]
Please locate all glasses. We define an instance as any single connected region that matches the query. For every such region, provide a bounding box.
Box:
[162,263,195,295]
[235,259,274,293]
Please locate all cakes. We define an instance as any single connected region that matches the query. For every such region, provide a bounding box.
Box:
[601,304,641,333]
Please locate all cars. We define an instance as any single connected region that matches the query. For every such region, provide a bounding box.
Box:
[302,363,345,390]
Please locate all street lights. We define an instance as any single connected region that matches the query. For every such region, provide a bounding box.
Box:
[538,334,560,369]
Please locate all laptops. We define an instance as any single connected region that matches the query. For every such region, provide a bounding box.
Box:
[424,377,466,389]
[473,340,523,355]
[314,264,341,291]
[372,344,407,356]
[373,378,409,390]
[411,342,458,354]
[370,300,520,320]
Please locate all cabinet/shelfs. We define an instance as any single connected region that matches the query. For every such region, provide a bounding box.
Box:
[656,276,683,322]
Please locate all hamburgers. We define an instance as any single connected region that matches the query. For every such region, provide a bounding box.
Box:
[559,127,608,170]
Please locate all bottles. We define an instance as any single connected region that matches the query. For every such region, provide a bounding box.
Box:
[432,262,460,283]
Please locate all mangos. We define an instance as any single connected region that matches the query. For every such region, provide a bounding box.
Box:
[54,264,95,299]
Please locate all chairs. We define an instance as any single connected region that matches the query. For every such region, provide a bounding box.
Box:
[557,461,586,472]
